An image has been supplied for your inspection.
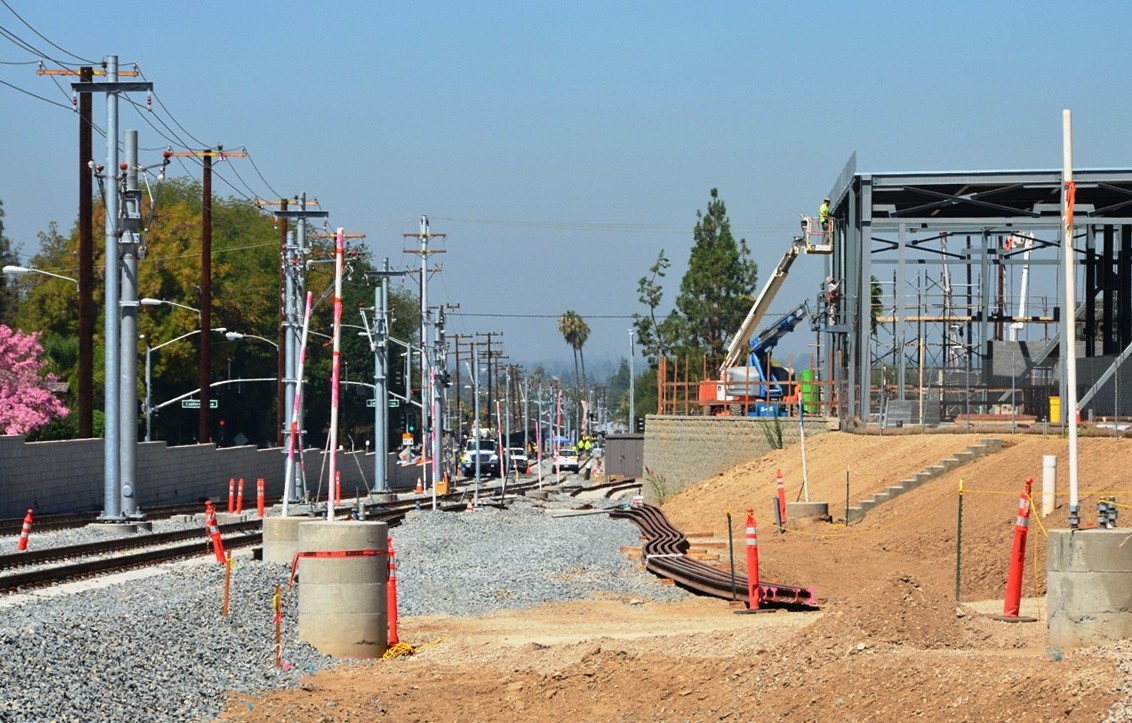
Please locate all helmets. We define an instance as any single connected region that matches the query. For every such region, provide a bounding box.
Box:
[825,277,833,283]
[824,198,830,203]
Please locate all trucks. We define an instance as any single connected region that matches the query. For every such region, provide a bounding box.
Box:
[458,437,501,478]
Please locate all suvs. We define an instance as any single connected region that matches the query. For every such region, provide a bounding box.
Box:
[502,447,529,474]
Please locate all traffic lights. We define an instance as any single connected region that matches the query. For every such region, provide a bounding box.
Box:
[637,417,644,433]
[426,416,433,433]
[407,412,416,433]
[400,413,406,433]
[442,369,451,388]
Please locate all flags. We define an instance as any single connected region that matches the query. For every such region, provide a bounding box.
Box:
[580,400,590,435]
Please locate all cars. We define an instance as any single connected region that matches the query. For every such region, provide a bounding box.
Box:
[551,448,581,474]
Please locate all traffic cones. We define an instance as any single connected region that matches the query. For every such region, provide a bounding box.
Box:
[466,498,473,512]
[414,477,424,495]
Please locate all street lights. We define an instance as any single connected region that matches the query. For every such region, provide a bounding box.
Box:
[145,328,227,442]
[141,297,211,444]
[358,332,411,464]
[2,265,94,439]
[224,330,284,447]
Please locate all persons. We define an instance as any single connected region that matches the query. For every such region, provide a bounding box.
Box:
[826,276,839,325]
[819,197,831,245]
[526,438,591,460]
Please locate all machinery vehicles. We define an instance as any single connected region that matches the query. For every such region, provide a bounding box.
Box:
[696,210,831,417]
